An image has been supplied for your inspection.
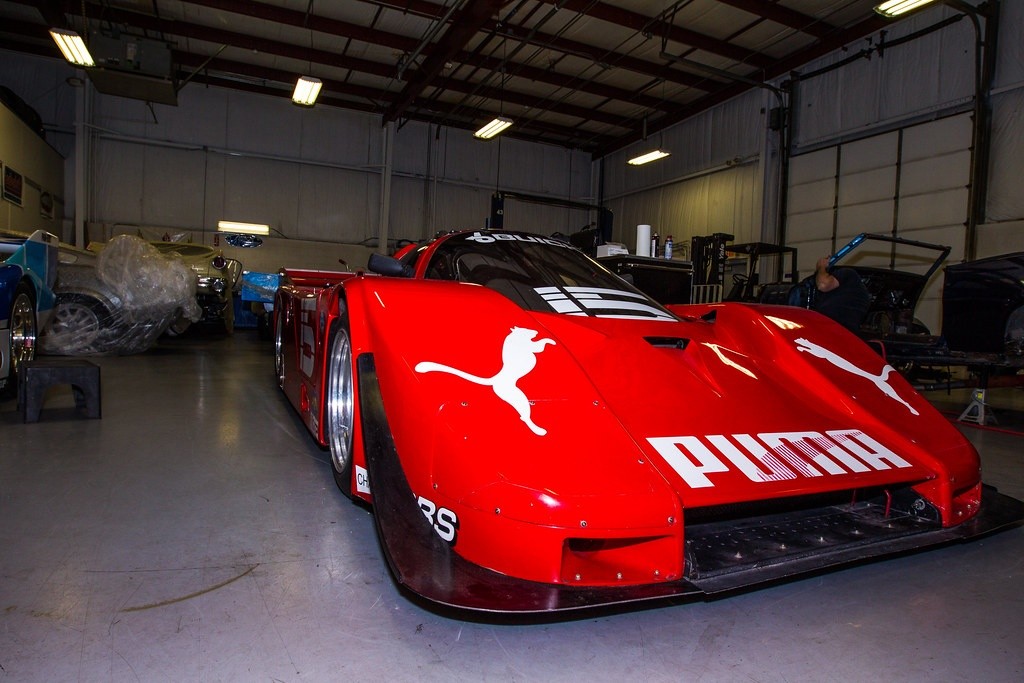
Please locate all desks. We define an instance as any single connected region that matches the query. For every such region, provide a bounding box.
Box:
[593,253,694,305]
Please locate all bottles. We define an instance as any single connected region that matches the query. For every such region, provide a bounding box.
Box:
[664,235,673,260]
[651,231,660,257]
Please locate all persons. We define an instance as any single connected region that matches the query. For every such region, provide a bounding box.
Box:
[810,255,887,335]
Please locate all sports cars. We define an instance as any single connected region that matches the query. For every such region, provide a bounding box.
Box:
[270,220,986,613]
[0,227,162,356]
[139,240,234,339]
